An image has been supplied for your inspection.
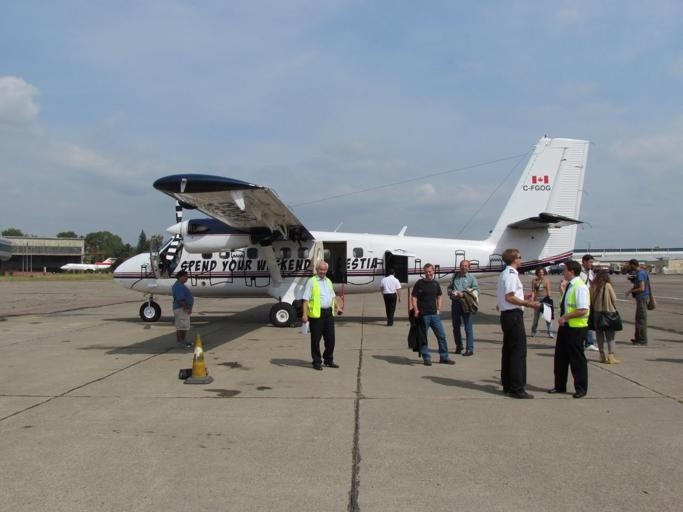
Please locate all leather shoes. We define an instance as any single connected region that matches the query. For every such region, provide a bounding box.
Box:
[324,357,340,369]
[572,390,588,398]
[312,361,323,371]
[549,387,567,393]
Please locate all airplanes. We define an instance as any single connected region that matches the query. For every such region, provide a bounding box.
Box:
[112,135,588,328]
[59,258,117,273]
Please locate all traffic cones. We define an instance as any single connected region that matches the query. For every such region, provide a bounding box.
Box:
[182,332,215,386]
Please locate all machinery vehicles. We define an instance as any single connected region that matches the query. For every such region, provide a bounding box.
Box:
[528,263,654,274]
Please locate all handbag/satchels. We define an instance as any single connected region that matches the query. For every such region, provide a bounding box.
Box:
[598,310,623,332]
[584,311,599,330]
[646,295,657,311]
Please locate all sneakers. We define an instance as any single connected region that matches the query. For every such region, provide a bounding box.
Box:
[176,339,194,348]
[583,344,599,351]
[423,354,432,365]
[438,356,454,364]
[509,390,535,400]
[600,353,622,365]
[456,346,464,353]
[631,336,648,346]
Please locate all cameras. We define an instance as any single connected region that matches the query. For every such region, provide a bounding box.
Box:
[627,275,636,281]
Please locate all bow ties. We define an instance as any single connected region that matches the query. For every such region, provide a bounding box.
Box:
[530,328,535,337]
[463,351,474,356]
[547,332,554,338]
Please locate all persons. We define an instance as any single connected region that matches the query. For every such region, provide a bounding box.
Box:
[547,260,591,398]
[301,260,343,370]
[624,258,651,346]
[495,248,543,396]
[447,260,480,356]
[577,255,599,351]
[171,270,195,345]
[530,265,555,339]
[410,263,456,366]
[588,269,623,364]
[379,268,403,325]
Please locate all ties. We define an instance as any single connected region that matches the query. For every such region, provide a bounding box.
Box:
[559,283,571,316]
